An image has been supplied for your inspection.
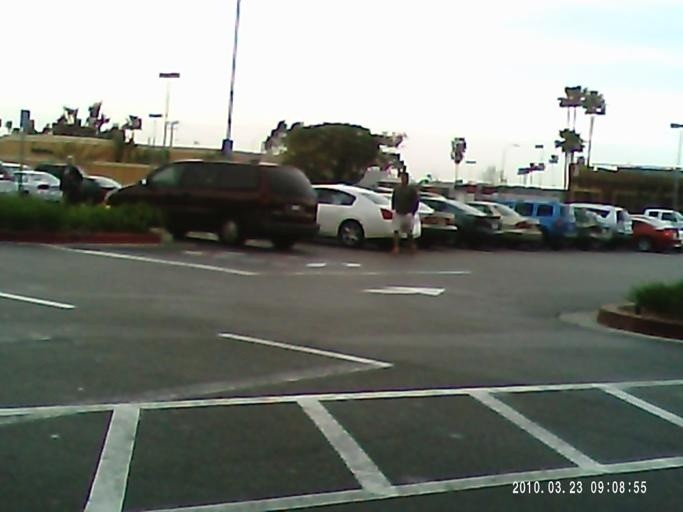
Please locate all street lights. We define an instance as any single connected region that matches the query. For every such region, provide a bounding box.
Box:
[149,73,180,159]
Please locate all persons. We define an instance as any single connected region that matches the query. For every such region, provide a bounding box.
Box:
[58,154,83,207]
[390,171,420,255]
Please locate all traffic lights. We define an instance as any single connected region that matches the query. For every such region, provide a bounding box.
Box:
[450,138,466,163]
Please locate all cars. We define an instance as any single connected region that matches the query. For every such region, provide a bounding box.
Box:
[367,186,683,252]
[310,183,422,249]
[0,160,124,206]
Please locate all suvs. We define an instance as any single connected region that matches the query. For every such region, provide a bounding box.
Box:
[103,160,321,249]
